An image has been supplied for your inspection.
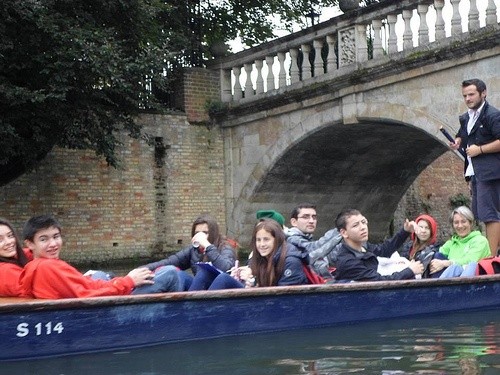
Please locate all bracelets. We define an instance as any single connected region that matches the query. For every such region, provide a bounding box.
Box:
[480,146,484,154]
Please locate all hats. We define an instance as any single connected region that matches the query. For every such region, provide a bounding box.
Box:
[256,209,285,227]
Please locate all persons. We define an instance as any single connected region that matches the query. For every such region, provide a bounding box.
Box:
[447,79,500,256]
[135,216,237,292]
[189,218,310,291]
[15,215,184,300]
[428,205,492,279]
[0,216,111,298]
[334,208,424,282]
[288,204,344,280]
[398,214,438,278]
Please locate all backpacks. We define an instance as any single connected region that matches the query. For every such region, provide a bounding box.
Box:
[476,255,500,276]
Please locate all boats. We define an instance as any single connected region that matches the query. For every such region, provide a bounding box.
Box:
[0,271,500,362]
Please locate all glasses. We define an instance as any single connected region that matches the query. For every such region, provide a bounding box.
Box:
[297,214,317,220]
